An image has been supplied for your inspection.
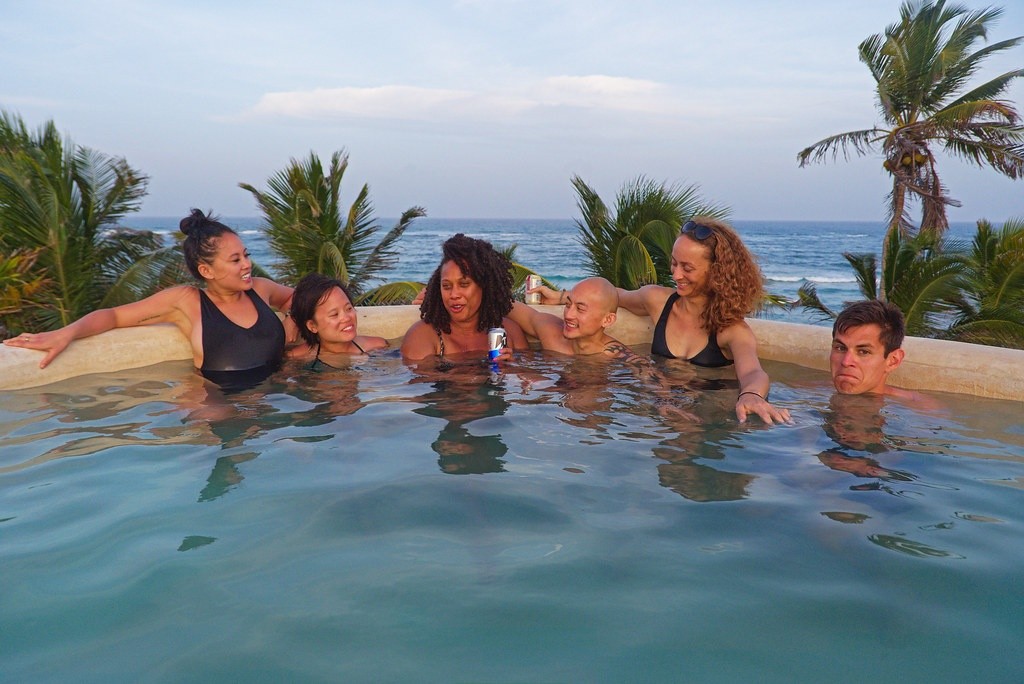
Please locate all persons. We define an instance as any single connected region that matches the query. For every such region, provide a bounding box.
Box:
[286,275,390,372]
[526,215,794,425]
[399,231,531,365]
[411,276,703,423]
[828,300,925,400]
[1,207,298,388]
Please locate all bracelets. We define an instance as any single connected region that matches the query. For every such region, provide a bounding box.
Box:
[559,289,566,304]
[737,391,764,400]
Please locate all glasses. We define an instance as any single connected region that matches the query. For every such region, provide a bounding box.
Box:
[681,221,715,240]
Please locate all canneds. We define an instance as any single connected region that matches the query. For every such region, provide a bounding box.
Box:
[488,327,507,363]
[525,275,542,305]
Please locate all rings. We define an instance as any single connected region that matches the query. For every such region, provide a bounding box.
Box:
[25,338,29,342]
